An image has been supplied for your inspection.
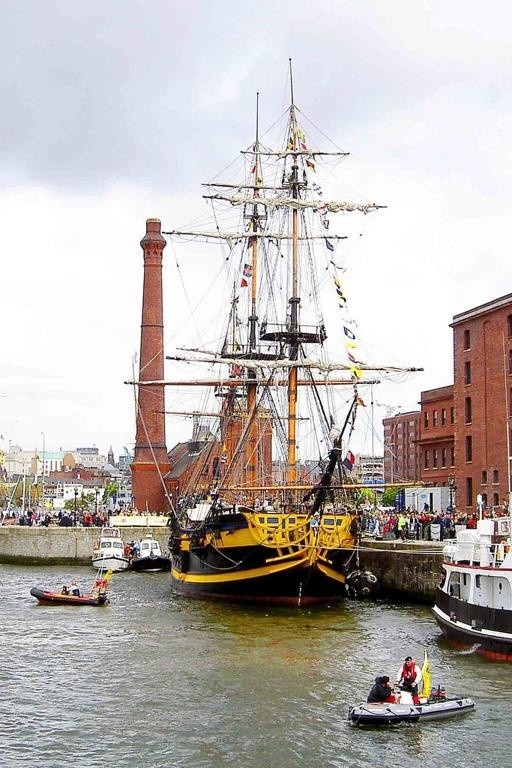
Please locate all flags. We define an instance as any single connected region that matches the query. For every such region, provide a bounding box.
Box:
[237,161,262,288]
[294,122,367,414]
[342,452,355,472]
[421,649,430,699]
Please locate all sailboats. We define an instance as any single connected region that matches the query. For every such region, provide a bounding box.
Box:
[122,57,424,608]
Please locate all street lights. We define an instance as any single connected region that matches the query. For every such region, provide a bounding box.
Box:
[8,439,12,485]
[40,432,45,513]
[74,485,98,524]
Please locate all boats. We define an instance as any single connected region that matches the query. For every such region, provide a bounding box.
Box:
[348,502,512,729]
[30,527,172,606]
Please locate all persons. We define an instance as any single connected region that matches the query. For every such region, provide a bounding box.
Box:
[126,540,135,548]
[71,581,83,597]
[59,585,71,596]
[328,495,510,539]
[29,508,173,527]
[365,673,393,704]
[392,653,424,705]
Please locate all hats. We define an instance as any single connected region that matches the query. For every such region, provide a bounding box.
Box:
[405,656,412,661]
